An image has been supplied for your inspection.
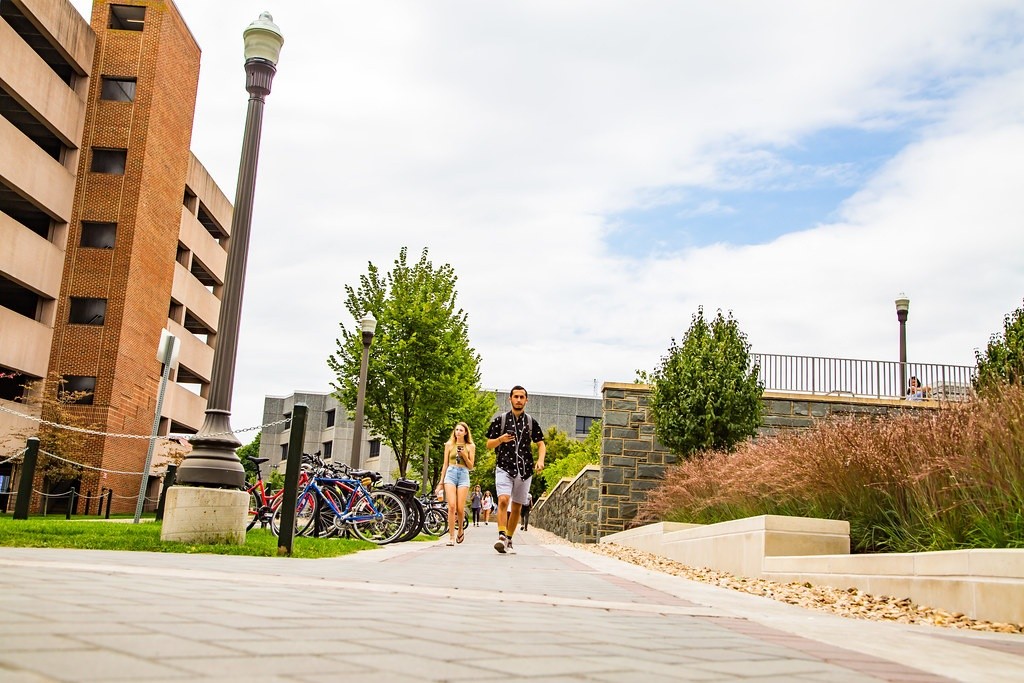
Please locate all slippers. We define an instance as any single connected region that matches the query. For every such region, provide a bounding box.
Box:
[456,530,464,543]
[446,542,454,546]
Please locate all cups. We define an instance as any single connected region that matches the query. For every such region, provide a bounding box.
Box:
[437,490,444,502]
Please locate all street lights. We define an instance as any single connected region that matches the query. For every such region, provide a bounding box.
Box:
[351,311,378,480]
[895,291,910,401]
[176,12,284,488]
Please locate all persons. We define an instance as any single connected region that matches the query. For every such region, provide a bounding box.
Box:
[484,385,547,554]
[483,491,495,525]
[907,377,931,400]
[494,501,497,513]
[470,485,484,527]
[507,492,532,531]
[435,422,476,546]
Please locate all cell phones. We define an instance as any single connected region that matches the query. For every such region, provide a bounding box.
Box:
[507,430,515,436]
[458,446,463,450]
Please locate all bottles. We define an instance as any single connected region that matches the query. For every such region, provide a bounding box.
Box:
[335,486,347,504]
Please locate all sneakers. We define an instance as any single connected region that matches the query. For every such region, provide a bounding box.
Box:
[494,535,506,553]
[507,541,515,553]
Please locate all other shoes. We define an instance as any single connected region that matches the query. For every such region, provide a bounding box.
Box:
[477,523,479,527]
[524,530,527,531]
[486,522,488,525]
[521,526,524,530]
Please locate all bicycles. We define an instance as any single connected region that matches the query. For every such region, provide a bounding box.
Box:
[240,450,469,545]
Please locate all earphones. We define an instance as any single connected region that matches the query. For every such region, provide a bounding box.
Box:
[526,402,527,404]
[510,400,512,404]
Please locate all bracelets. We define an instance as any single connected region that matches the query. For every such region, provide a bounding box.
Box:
[499,437,501,443]
